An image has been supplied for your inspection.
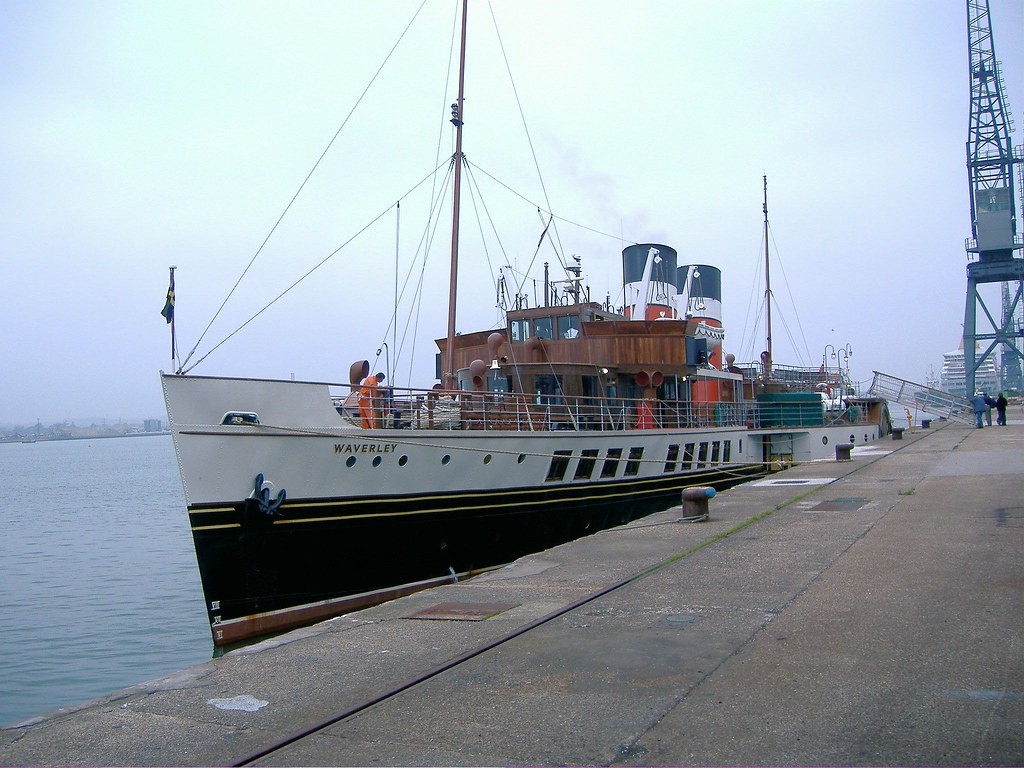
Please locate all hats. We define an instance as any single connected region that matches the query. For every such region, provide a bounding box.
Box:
[997,392,1003,397]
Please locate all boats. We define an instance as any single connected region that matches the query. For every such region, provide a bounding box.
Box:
[155,0,893,646]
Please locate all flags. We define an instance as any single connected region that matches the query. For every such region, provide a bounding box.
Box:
[160,271,174,324]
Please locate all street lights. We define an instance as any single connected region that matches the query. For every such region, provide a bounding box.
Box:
[824,344,836,384]
[846,343,852,374]
[837,348,848,419]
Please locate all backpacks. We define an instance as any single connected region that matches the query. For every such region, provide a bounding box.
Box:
[986,398,997,408]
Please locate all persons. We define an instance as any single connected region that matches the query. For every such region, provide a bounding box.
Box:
[972,391,1008,428]
[357,372,385,430]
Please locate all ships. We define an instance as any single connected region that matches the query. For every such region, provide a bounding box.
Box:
[938,332,1002,407]
